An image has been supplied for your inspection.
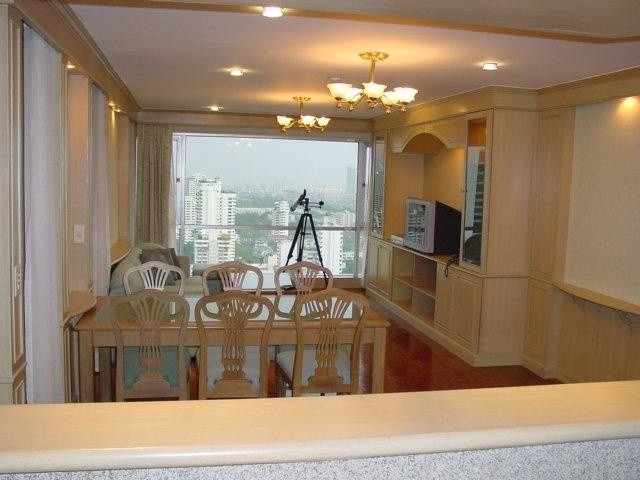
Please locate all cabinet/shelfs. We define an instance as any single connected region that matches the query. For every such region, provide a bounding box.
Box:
[370,130,424,239]
[365,236,529,368]
[458,108,537,276]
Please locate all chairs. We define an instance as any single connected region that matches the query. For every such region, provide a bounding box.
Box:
[110,260,371,402]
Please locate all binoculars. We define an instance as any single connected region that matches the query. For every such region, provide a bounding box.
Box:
[290,189,309,213]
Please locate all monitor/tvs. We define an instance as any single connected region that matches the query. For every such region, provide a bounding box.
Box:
[403,197,461,256]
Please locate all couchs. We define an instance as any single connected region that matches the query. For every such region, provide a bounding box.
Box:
[110,242,203,295]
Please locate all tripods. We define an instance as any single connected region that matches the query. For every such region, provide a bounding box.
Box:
[281,209,327,289]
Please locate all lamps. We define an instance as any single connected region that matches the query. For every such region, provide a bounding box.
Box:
[326,51,419,115]
[276,97,331,133]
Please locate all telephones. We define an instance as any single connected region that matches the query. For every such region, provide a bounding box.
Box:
[449,253,459,265]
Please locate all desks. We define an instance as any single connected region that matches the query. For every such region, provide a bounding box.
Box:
[73,295,391,403]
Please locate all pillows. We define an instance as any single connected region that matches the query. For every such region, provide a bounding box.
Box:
[139,248,181,286]
